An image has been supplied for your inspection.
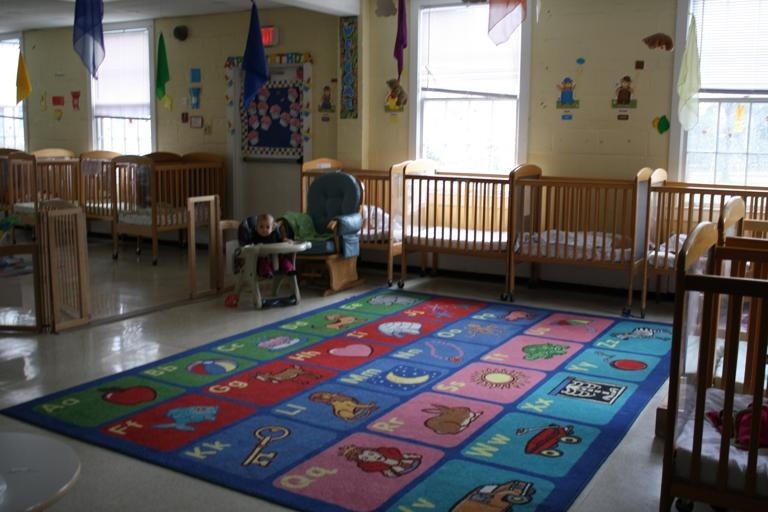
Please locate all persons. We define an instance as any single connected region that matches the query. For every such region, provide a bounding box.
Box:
[320,86,332,109]
[252,213,297,278]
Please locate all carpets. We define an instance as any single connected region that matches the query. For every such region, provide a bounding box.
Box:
[1,288,673,512]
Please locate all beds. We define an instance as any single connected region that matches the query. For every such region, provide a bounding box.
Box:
[0,146,224,266]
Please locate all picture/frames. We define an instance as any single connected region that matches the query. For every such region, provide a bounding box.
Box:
[238,79,303,161]
